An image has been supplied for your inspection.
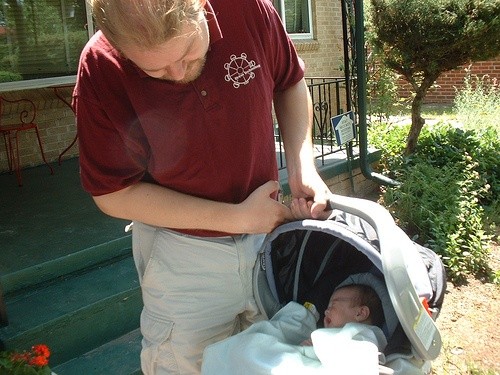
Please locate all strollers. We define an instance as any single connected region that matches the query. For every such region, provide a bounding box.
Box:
[201,193,446,375]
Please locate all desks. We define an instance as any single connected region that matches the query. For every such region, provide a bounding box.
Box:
[46,85,77,166]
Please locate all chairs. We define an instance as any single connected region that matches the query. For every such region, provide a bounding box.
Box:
[0,96,54,187]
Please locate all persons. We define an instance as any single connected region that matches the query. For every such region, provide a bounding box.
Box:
[200,282,385,375]
[72,0,333,375]
